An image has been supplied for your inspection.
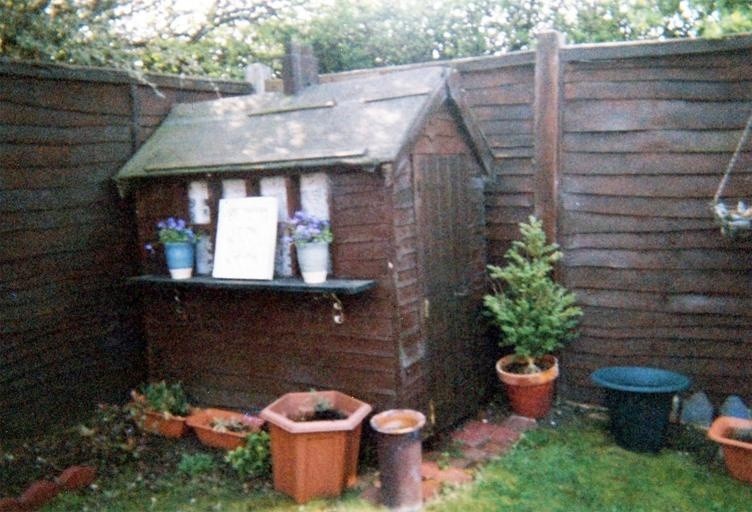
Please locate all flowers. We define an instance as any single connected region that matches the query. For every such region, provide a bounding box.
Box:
[280,210,338,245]
[141,214,198,252]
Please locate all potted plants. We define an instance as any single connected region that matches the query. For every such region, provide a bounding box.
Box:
[122,380,191,448]
[261,387,371,503]
[185,403,261,456]
[479,211,587,426]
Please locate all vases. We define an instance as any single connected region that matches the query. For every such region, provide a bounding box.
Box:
[366,409,427,512]
[591,363,692,457]
[292,240,327,283]
[166,242,194,278]
[707,413,752,481]
